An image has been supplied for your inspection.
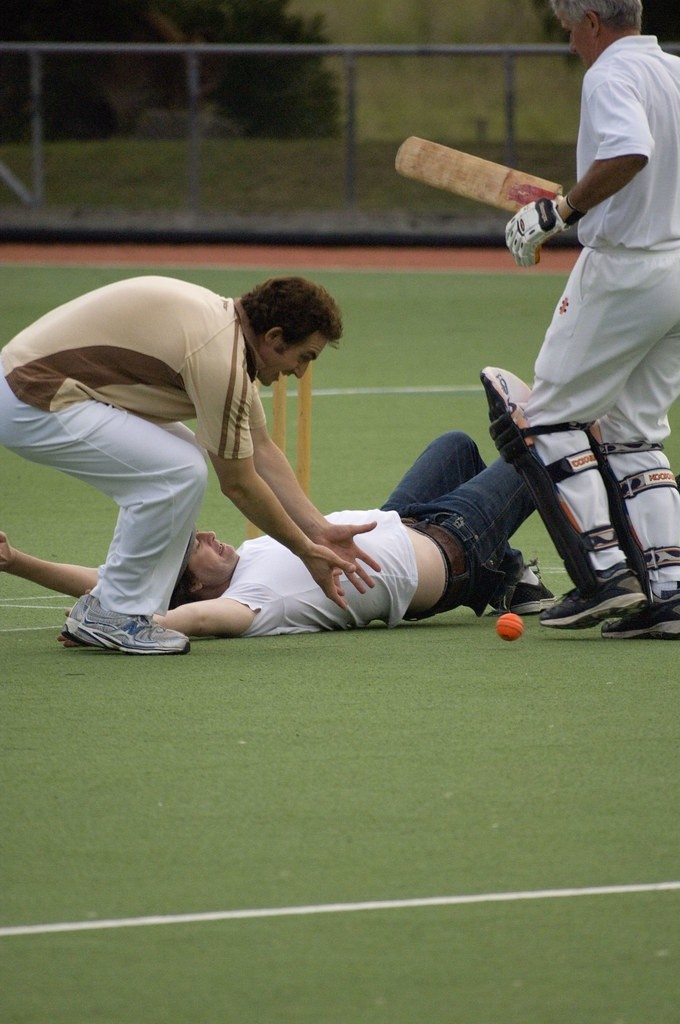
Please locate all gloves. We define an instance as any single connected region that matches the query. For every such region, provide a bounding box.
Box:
[504,197,570,268]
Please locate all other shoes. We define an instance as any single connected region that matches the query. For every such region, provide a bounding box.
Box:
[510,575,556,614]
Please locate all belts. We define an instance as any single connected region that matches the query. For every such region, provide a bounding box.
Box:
[401,518,464,619]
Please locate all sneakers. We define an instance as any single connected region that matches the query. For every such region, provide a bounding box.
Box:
[60,594,95,642]
[601,591,680,639]
[72,598,190,655]
[539,568,648,629]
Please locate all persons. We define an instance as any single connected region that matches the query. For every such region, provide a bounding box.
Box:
[1,276,381,654]
[504,0,679,638]
[0,432,550,646]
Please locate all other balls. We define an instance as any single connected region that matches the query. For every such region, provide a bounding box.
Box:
[495,612,526,642]
